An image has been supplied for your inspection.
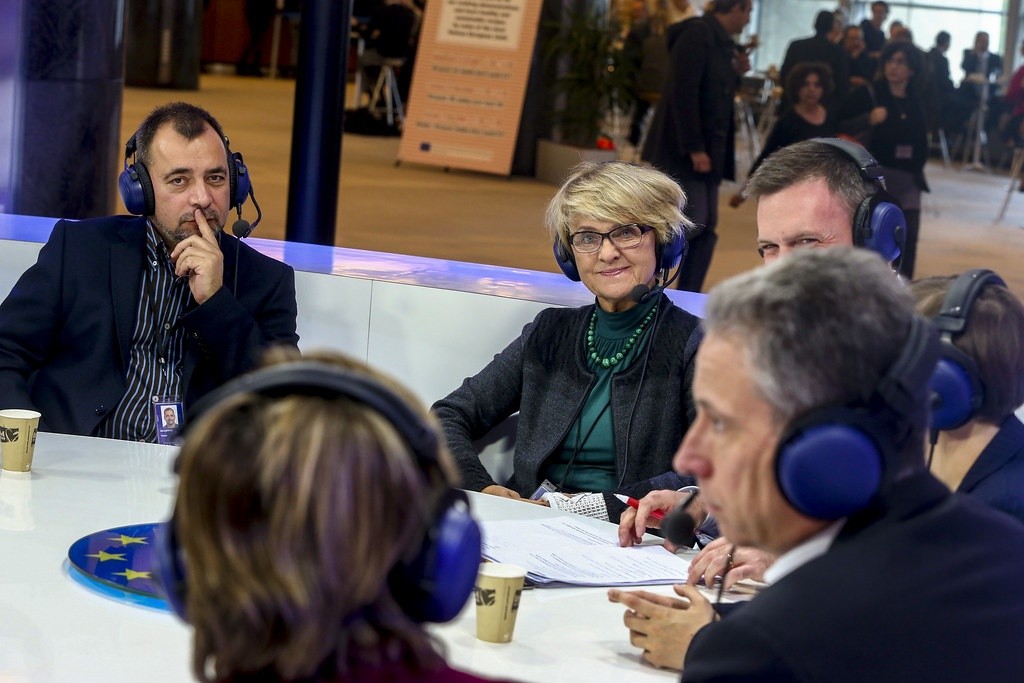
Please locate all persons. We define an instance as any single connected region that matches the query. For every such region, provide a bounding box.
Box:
[428,161,706,522]
[169,352,505,683]
[611,268,1024,673]
[0,101,299,447]
[601,0,1024,290]
[163,408,178,429]
[237,0,275,75]
[673,245,1024,683]
[364,0,415,104]
[617,137,910,589]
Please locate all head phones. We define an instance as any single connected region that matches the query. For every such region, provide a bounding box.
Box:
[118,131,250,216]
[815,137,907,262]
[147,362,482,623]
[924,268,1008,445]
[773,312,938,520]
[553,160,685,282]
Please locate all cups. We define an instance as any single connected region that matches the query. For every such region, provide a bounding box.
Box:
[0,469,36,529]
[0,408,42,473]
[473,562,528,643]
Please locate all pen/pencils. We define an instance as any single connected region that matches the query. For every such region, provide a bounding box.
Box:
[612,493,664,519]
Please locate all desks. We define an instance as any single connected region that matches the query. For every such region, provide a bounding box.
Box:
[0,411,692,683]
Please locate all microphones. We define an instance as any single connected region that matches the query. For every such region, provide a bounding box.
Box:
[630,241,689,304]
[660,510,705,551]
[232,184,262,238]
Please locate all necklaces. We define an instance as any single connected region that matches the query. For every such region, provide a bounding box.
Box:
[588,304,660,366]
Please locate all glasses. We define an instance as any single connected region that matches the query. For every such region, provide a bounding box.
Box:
[569,224,653,253]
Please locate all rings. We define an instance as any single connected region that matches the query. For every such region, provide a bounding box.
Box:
[726,552,735,569]
